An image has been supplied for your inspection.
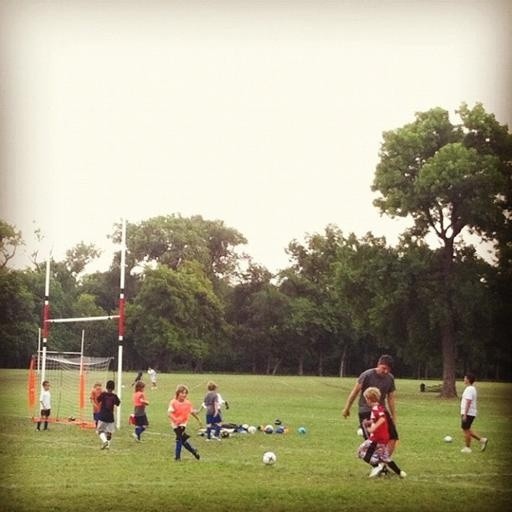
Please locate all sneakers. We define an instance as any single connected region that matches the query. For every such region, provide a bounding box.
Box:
[205,438,212,442]
[173,457,180,460]
[212,435,221,441]
[369,463,382,478]
[399,471,407,477]
[193,449,200,460]
[460,447,472,453]
[479,437,488,451]
[100,441,109,450]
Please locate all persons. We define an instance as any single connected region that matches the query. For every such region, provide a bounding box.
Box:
[95,380,120,450]
[195,381,224,442]
[35,381,51,432]
[167,384,200,461]
[342,355,399,458]
[357,386,407,479]
[89,384,103,428]
[131,366,158,443]
[461,375,488,453]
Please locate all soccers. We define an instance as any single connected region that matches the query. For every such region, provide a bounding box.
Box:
[265,425,273,434]
[276,427,284,434]
[263,452,276,465]
[357,428,363,436]
[258,425,265,432]
[298,427,306,435]
[445,436,452,443]
[68,415,75,422]
[274,419,281,425]
